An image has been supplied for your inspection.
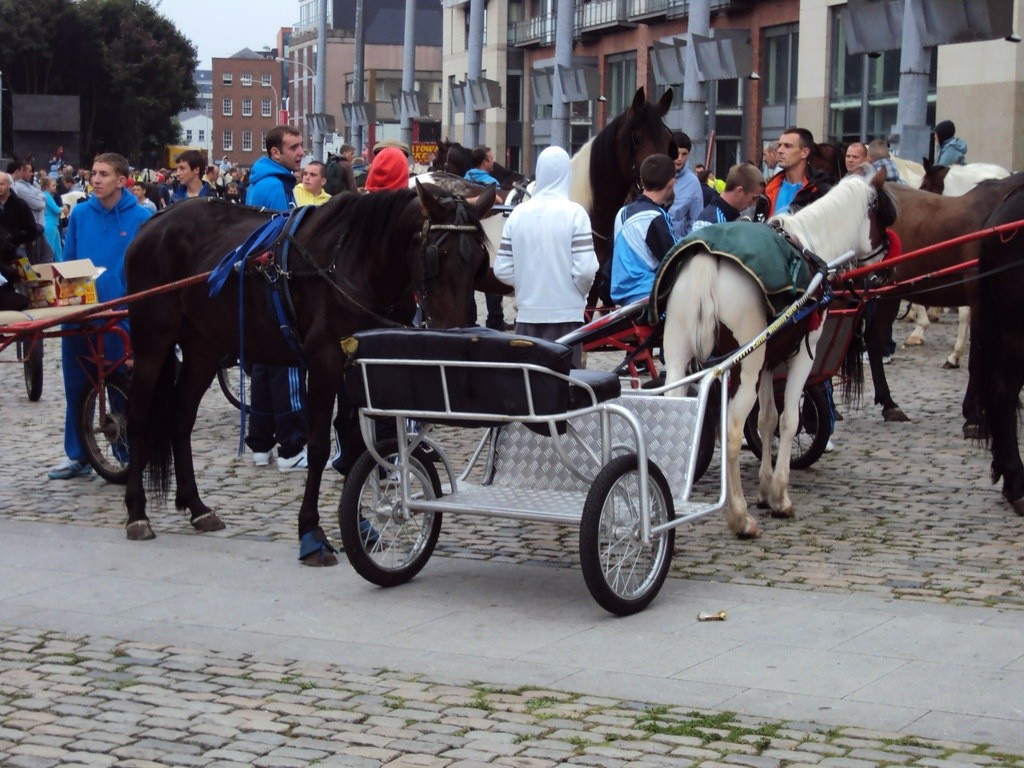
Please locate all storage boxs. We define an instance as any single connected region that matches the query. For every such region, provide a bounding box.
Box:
[28,257,106,305]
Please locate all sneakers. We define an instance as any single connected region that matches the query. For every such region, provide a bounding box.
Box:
[253,450,273,467]
[47,458,93,479]
[277,449,332,472]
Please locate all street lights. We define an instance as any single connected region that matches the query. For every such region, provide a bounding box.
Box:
[240,78,278,126]
[275,57,316,112]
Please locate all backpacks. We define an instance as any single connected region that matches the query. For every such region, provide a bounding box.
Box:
[323,158,346,195]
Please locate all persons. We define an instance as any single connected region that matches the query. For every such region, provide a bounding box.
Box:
[611,154,678,326]
[48,152,172,481]
[464,146,511,332]
[2,119,1024,481]
[245,125,337,472]
[494,146,601,375]
[935,120,967,167]
[165,150,220,205]
[760,127,834,453]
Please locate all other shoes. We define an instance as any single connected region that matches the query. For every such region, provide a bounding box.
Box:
[823,439,834,453]
[881,354,893,364]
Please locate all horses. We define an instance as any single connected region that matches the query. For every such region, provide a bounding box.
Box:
[842,172,1024,423]
[125,178,497,567]
[865,145,1010,369]
[971,185,1023,517]
[663,164,888,538]
[428,137,523,190]
[409,86,679,331]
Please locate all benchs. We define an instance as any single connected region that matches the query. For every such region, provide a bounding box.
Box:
[341,329,621,424]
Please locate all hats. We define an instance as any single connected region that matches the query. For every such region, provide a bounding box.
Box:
[932,120,955,149]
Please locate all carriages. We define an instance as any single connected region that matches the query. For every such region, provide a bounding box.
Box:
[0,178,498,568]
[350,84,679,339]
[339,155,897,616]
[556,183,1024,518]
